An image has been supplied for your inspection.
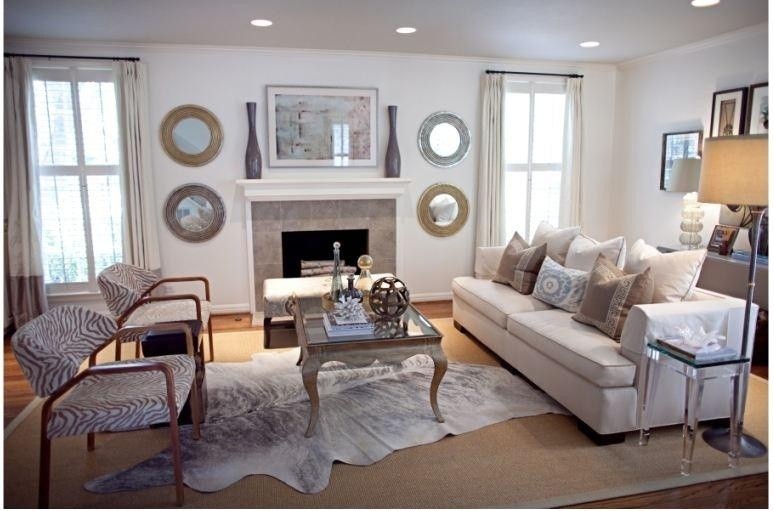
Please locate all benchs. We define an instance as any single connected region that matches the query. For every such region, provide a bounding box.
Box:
[263,273,403,348]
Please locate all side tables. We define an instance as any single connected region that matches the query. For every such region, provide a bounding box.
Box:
[141,320,208,429]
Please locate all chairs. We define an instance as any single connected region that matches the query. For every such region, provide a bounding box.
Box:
[97,262,214,361]
[11,304,201,509]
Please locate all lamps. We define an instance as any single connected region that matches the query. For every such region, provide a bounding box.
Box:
[679,192,705,250]
[698,135,768,459]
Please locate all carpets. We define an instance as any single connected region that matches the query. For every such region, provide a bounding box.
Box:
[4,317,768,509]
[83,347,572,494]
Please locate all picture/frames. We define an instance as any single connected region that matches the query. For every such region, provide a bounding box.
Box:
[266,85,379,168]
[660,82,768,190]
[707,225,740,254]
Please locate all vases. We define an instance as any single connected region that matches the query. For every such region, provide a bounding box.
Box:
[386,106,400,178]
[246,102,262,179]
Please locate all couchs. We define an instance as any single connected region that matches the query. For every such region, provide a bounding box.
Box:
[452,247,760,445]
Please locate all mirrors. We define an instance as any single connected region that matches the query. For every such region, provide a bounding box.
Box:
[417,183,470,237]
[163,182,227,243]
[418,112,472,168]
[159,104,225,167]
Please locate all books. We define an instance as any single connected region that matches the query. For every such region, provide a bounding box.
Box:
[655,325,736,365]
[321,306,375,342]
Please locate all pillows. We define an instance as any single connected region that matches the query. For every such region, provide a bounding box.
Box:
[492,220,707,343]
